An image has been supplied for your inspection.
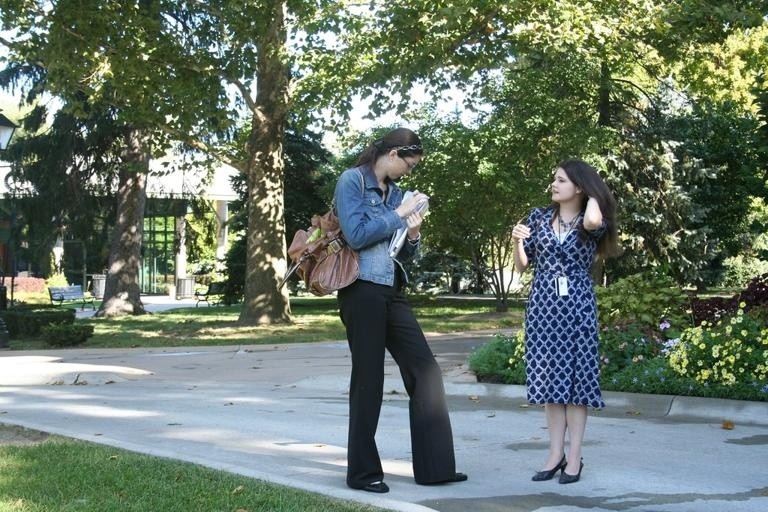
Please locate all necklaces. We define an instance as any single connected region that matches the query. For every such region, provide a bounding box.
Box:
[557,214,576,234]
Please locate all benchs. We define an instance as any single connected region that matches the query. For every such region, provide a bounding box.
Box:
[48,286,97,311]
[195,282,223,307]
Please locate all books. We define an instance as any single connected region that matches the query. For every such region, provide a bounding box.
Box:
[387,189,430,260]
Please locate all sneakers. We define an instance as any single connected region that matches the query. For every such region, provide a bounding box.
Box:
[447,472,468,482]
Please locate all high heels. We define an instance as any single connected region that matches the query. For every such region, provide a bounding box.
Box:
[531,452,567,482]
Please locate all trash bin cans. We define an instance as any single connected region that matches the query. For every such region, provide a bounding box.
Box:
[177,278,195,298]
[92,274,107,299]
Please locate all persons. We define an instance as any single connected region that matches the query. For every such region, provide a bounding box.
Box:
[508,156,624,486]
[328,125,466,494]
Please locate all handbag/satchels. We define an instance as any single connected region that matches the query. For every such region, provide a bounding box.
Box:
[278,168,366,297]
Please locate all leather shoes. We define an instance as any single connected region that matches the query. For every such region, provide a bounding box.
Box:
[558,459,585,484]
[362,481,390,493]
